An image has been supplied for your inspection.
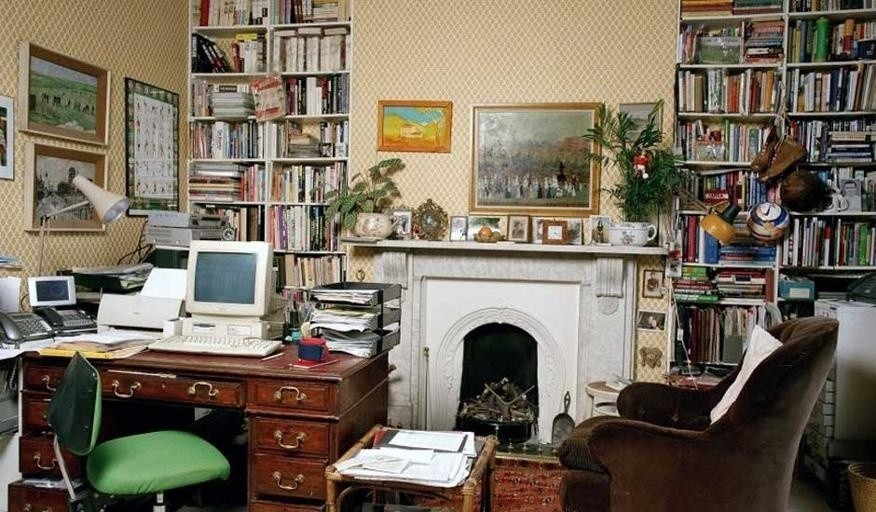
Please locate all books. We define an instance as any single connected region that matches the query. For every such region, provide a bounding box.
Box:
[285,75,350,113]
[192,80,254,118]
[681,217,775,264]
[786,62,876,114]
[678,118,772,164]
[679,68,781,114]
[269,119,347,158]
[677,307,764,378]
[189,163,265,202]
[269,206,346,250]
[190,122,264,158]
[270,28,350,72]
[789,167,875,213]
[681,0,783,16]
[191,0,268,27]
[673,269,772,303]
[194,206,265,241]
[270,1,351,24]
[790,0,875,12]
[783,217,876,266]
[787,15,874,61]
[681,169,775,213]
[192,31,267,73]
[791,120,875,161]
[268,162,348,203]
[682,22,784,66]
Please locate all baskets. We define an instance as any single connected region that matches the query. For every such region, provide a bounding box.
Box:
[847,460,875,510]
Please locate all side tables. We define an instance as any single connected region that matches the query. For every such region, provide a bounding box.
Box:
[323,423,498,512]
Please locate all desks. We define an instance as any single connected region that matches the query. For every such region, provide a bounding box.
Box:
[6,335,396,512]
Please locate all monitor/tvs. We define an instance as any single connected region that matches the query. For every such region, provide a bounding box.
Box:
[184,239,277,324]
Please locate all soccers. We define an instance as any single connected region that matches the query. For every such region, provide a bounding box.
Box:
[748,201,791,242]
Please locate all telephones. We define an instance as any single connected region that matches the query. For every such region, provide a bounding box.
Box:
[846,272,876,304]
[34,306,98,336]
[0,312,53,349]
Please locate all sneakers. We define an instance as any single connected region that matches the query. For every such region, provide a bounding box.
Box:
[751,125,779,172]
[756,135,808,183]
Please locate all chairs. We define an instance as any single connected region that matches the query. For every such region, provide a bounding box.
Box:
[45,348,231,512]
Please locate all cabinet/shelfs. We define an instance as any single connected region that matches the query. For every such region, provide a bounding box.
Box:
[185,0,353,291]
[665,0,876,383]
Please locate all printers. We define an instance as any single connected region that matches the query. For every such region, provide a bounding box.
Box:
[95,267,187,337]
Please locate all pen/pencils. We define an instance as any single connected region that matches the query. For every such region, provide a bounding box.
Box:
[260,351,286,363]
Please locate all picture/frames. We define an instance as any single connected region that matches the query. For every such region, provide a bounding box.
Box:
[637,309,667,332]
[567,218,583,245]
[589,215,611,245]
[0,94,15,181]
[390,204,415,240]
[642,269,665,299]
[543,220,568,244]
[16,41,113,147]
[22,141,110,233]
[449,216,467,242]
[376,100,452,154]
[467,102,605,218]
[411,198,449,242]
[466,215,509,243]
[506,214,532,243]
[619,102,663,143]
[531,216,544,245]
[123,75,181,219]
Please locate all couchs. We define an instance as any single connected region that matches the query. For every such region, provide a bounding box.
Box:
[556,314,841,512]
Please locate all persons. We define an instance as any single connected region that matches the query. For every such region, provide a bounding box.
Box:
[647,273,661,291]
[596,220,604,242]
[643,316,659,330]
[841,181,861,211]
[512,221,523,239]
[482,171,587,202]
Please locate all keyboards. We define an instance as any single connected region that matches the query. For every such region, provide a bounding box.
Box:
[148,334,283,358]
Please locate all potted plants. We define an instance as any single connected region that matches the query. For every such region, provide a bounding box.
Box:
[579,97,694,249]
[308,158,405,241]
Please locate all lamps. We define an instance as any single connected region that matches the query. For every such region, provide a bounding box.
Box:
[36,173,131,276]
[677,185,742,246]
